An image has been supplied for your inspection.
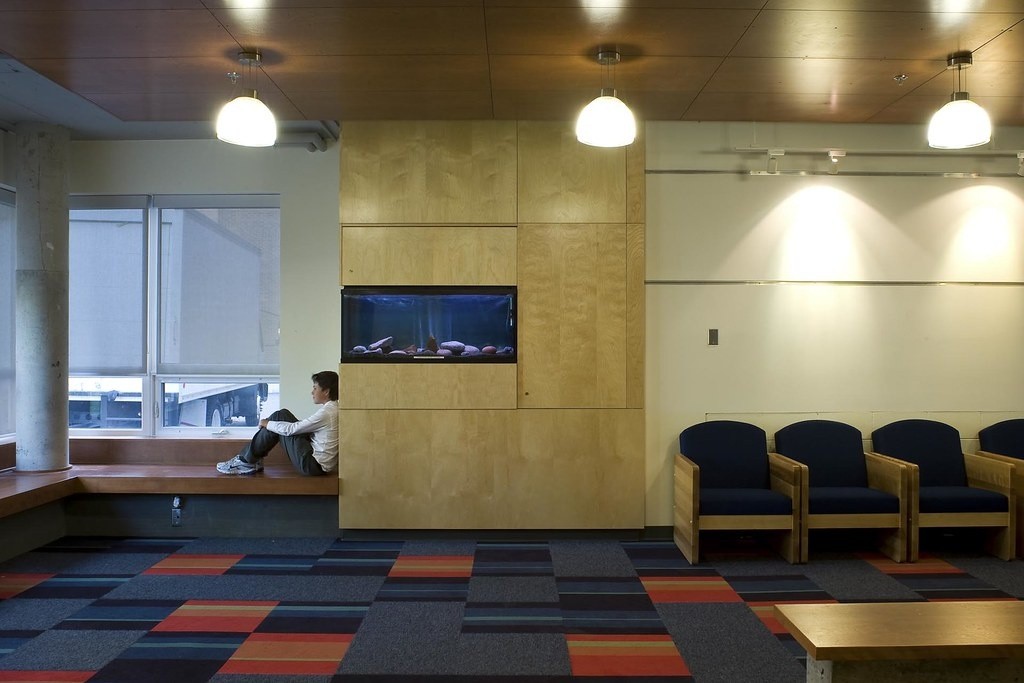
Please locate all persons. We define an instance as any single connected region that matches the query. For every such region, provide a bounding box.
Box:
[215,370,339,478]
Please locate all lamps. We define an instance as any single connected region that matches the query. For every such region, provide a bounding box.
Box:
[926,55,993,149]
[574,51,638,148]
[765,150,785,174]
[214,53,277,148]
[827,151,846,176]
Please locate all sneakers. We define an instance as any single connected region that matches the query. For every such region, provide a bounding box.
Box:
[216,455,256,475]
[256,459,264,472]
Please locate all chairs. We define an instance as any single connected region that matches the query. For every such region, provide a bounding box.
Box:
[773,420,908,564]
[871,419,1018,562]
[673,421,800,567]
[976,419,1024,559]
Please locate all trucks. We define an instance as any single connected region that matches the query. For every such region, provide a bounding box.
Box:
[68,382,269,427]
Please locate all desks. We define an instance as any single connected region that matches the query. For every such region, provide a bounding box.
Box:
[772,601,1024,683]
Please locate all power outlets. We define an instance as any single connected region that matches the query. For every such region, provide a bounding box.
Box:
[172,509,182,528]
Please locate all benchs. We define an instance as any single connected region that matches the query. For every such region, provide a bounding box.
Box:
[0,438,339,565]
